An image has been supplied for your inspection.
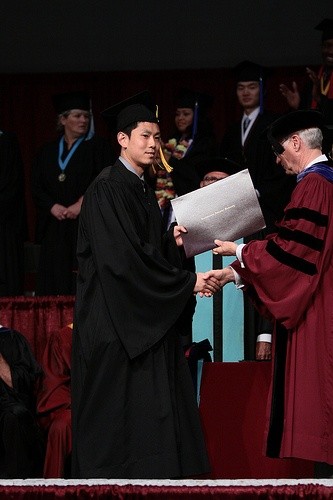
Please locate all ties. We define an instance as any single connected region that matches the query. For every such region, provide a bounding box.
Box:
[243,118,250,134]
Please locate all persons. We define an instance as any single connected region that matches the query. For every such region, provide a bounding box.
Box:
[71,104,221,479]
[30,88,116,296]
[153,17,333,362]
[202,110,333,479]
[1,323,43,479]
[39,324,80,478]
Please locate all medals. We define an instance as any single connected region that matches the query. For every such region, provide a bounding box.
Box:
[58,173,66,182]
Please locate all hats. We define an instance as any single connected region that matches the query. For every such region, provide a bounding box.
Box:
[263,109,324,154]
[99,88,174,174]
[175,88,214,136]
[226,60,269,115]
[51,87,94,142]
